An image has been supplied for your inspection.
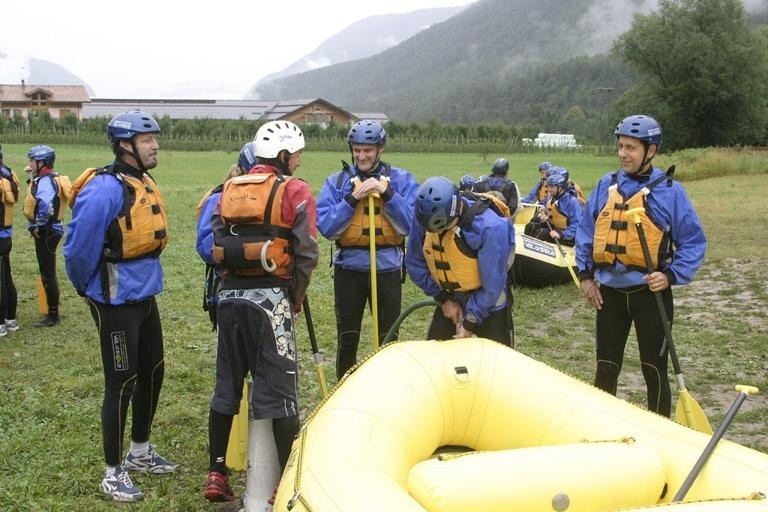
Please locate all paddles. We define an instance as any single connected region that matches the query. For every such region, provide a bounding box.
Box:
[625,207,714,438]
[226,380,251,471]
[26,169,51,315]
[303,294,328,401]
[547,222,583,288]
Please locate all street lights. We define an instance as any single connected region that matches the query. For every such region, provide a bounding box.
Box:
[597,86,616,157]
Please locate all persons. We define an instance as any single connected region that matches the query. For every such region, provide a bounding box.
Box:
[24,146,65,327]
[574,115,708,418]
[63,110,179,502]
[0,145,19,337]
[460,158,587,247]
[202,120,320,504]
[316,120,422,382]
[196,141,273,324]
[405,175,517,348]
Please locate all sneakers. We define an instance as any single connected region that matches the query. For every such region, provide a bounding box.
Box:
[35,317,59,327]
[0,318,19,337]
[100,467,143,502]
[204,471,234,501]
[125,445,179,474]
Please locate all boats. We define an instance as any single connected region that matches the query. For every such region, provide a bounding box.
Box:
[273,334,768,512]
[509,201,581,287]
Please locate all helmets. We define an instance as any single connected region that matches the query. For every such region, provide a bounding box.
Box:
[460,175,475,188]
[108,111,160,153]
[614,115,662,145]
[347,120,386,144]
[415,177,460,233]
[238,120,306,170]
[27,145,55,168]
[539,162,568,187]
[493,158,508,173]
